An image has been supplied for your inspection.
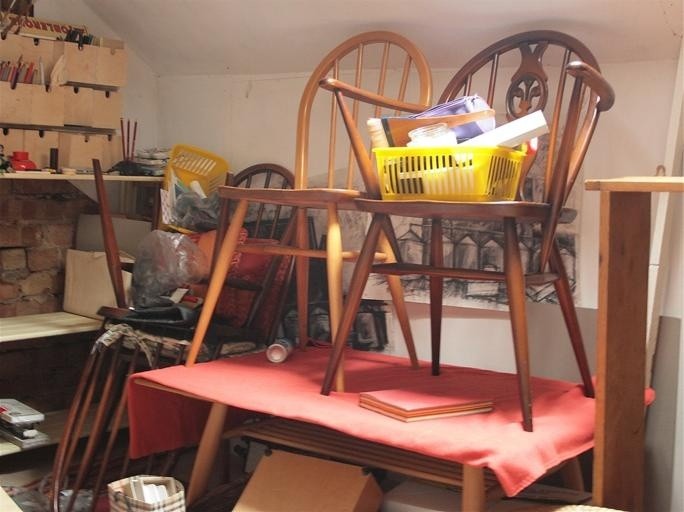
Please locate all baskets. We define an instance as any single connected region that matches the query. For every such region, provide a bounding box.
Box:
[372,146,528,203]
[157,143,231,233]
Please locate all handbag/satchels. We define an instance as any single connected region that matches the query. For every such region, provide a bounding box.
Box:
[62,249,136,323]
[107,473,188,512]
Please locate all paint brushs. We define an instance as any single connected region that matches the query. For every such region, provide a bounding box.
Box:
[120,117,137,160]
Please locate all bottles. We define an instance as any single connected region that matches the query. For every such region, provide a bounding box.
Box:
[267,338,294,363]
[9,151,36,171]
[404,123,457,149]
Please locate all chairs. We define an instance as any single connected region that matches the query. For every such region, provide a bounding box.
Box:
[186,29,432,392]
[319,30,615,429]
[39,151,302,512]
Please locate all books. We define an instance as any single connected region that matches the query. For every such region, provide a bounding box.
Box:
[359,389,493,423]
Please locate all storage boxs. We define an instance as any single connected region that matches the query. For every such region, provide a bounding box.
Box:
[229,447,384,512]
[1,22,129,173]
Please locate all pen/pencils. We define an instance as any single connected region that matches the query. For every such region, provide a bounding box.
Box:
[64,28,93,44]
[0,54,44,89]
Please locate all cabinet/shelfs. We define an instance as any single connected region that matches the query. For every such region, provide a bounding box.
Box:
[0,166,168,457]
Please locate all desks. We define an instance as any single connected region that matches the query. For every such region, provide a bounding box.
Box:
[127,343,597,512]
